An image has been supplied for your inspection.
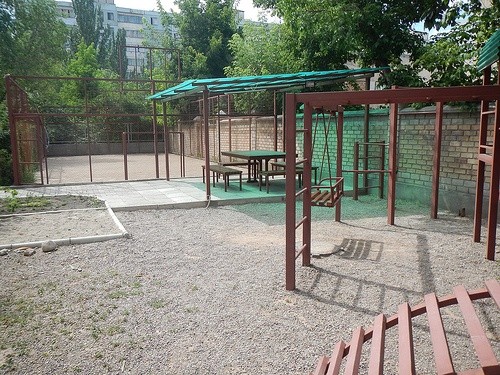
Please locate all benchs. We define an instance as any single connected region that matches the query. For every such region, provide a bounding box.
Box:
[217,160,260,180]
[201,164,243,192]
[310,177,344,207]
[257,169,304,194]
[271,161,319,186]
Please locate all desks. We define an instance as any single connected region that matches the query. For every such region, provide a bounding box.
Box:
[221,149,298,194]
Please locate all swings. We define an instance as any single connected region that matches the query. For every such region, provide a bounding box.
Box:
[311,111,344,208]
